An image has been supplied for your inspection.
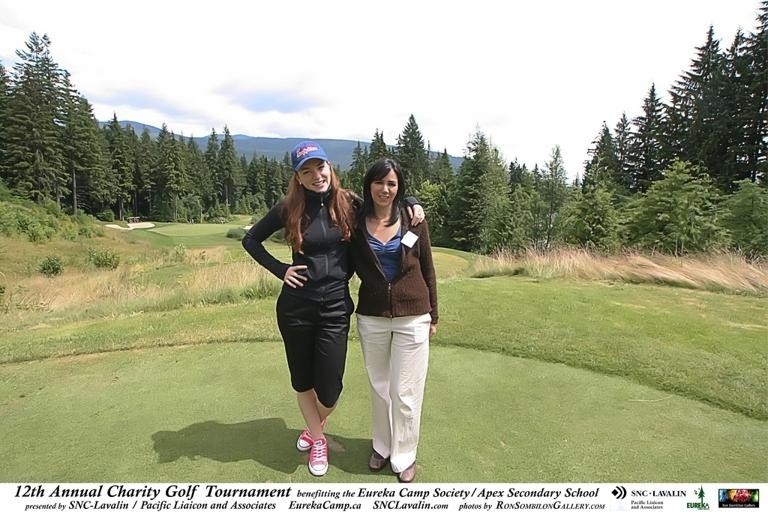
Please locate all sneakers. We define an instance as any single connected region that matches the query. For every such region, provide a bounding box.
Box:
[399,461,416,483]
[309,435,329,476]
[297,417,327,452]
[368,450,387,472]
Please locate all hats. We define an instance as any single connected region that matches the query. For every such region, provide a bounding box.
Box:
[290,141,329,173]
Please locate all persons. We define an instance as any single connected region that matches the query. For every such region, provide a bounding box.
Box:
[241,139,426,478]
[347,153,440,484]
